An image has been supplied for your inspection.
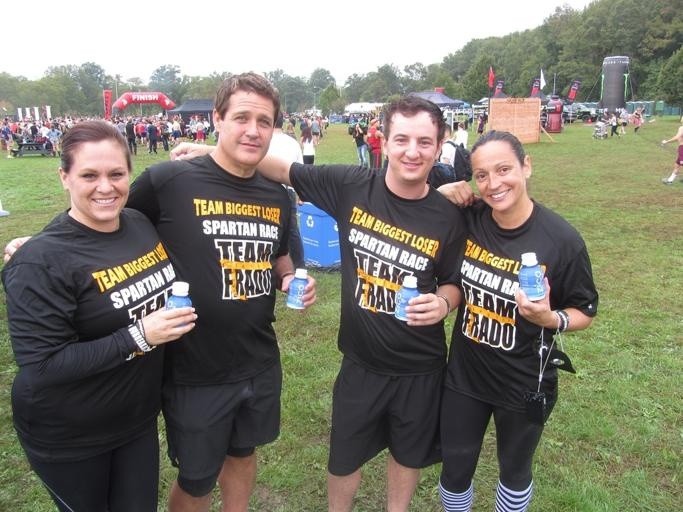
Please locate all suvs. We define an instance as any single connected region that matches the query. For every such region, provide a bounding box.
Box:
[347,112,367,135]
[536,100,599,123]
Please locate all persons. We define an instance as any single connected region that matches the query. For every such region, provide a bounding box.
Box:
[116,115,210,155]
[0,119,84,158]
[2,122,196,512]
[476,116,484,136]
[354,118,384,169]
[601,107,643,137]
[468,105,473,126]
[267,110,329,165]
[4,72,320,512]
[439,123,467,168]
[171,95,468,512]
[436,131,598,512]
[660,116,683,185]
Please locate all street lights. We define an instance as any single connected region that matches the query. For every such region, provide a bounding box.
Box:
[106,78,118,101]
[314,87,323,91]
[326,77,335,89]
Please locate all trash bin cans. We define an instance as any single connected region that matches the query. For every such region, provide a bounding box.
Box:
[297,203,342,273]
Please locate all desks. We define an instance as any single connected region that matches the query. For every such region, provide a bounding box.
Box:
[10,143,53,157]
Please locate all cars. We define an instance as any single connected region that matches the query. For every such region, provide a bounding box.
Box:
[159,121,191,136]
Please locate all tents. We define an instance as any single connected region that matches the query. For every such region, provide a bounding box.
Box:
[172,100,215,113]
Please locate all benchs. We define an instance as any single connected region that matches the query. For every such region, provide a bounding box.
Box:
[10,149,52,156]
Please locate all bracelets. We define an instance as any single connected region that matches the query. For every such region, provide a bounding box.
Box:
[278,270,294,287]
[128,321,152,353]
[438,294,450,319]
[557,309,570,336]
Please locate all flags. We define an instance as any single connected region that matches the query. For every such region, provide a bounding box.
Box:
[540,69,546,90]
[488,67,494,87]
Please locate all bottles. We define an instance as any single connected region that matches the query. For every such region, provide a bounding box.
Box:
[393,275,420,323]
[164,280,192,310]
[285,267,308,310]
[661,140,665,146]
[516,252,547,302]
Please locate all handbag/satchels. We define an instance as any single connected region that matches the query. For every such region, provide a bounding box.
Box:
[428,140,473,189]
